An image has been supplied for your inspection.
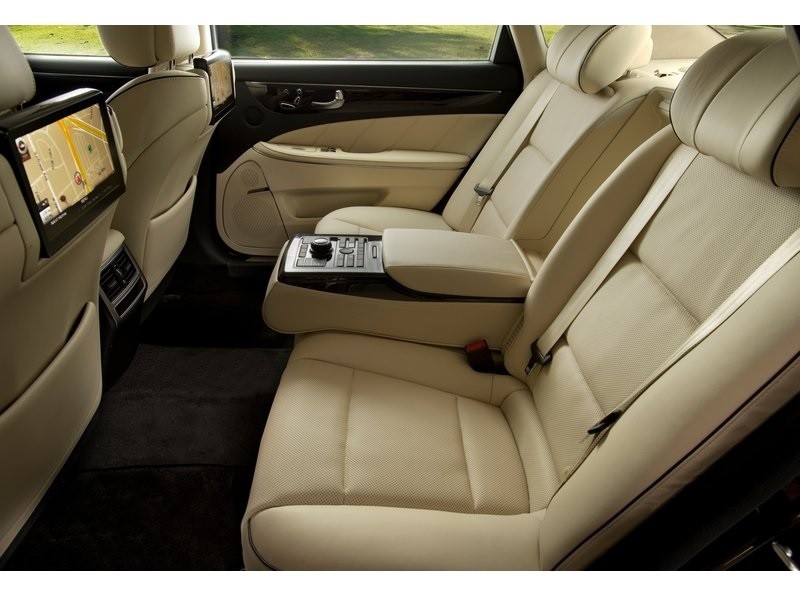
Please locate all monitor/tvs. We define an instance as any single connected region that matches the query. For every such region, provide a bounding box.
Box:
[0,85,129,258]
[194,48,238,125]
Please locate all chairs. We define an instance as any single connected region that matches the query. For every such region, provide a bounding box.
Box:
[315,25,665,269]
[239,31,800,571]
[1,26,128,567]
[95,25,232,302]
